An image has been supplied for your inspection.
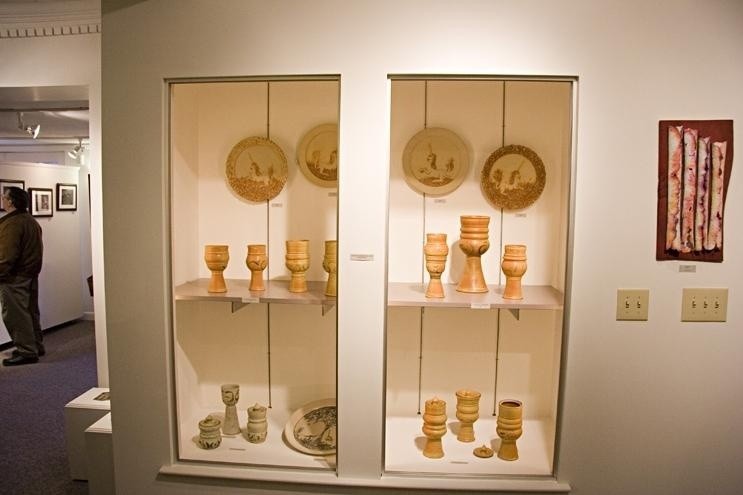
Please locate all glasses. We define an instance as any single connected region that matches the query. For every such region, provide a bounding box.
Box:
[2,194,8,200]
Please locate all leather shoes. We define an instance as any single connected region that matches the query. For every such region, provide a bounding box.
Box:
[3,348,46,366]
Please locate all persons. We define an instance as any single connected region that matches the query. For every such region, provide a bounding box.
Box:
[0,187,45,366]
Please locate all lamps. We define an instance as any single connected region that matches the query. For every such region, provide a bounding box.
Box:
[16,111,40,139]
[67,139,85,159]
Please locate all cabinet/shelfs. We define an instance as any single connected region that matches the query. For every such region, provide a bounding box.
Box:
[382,74,575,481]
[163,75,341,475]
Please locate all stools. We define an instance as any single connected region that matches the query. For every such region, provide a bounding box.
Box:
[60,387,112,494]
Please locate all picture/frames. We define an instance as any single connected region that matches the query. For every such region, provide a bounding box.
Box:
[0,178,78,218]
